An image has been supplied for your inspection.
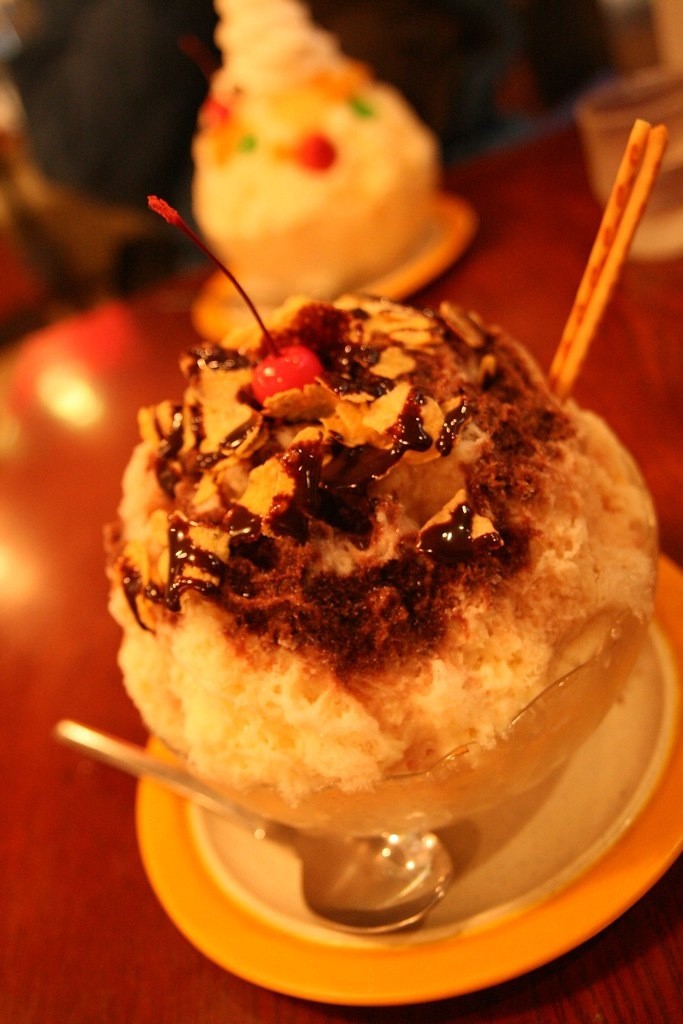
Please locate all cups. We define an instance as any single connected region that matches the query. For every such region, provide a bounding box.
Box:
[574,66,683,263]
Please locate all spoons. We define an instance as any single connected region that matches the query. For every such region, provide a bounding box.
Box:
[54,717,454,938]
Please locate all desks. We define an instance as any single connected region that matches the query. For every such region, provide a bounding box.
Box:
[1,114,683,1024]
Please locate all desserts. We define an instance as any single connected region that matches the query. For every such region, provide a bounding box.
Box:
[188,0,440,308]
[101,196,659,839]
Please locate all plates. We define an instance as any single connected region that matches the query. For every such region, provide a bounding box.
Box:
[132,551,683,1005]
[194,191,474,351]
